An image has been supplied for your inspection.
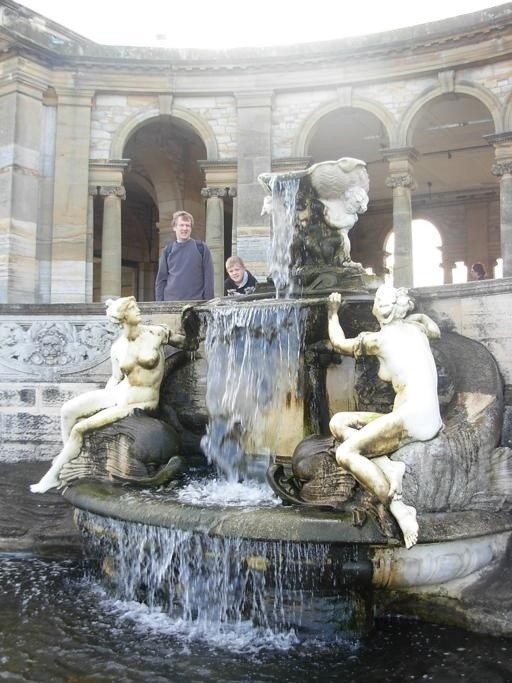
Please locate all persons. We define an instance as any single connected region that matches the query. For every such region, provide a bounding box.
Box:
[153,210,216,302]
[27,294,200,495]
[470,263,487,280]
[315,186,370,268]
[325,282,443,550]
[222,255,258,294]
